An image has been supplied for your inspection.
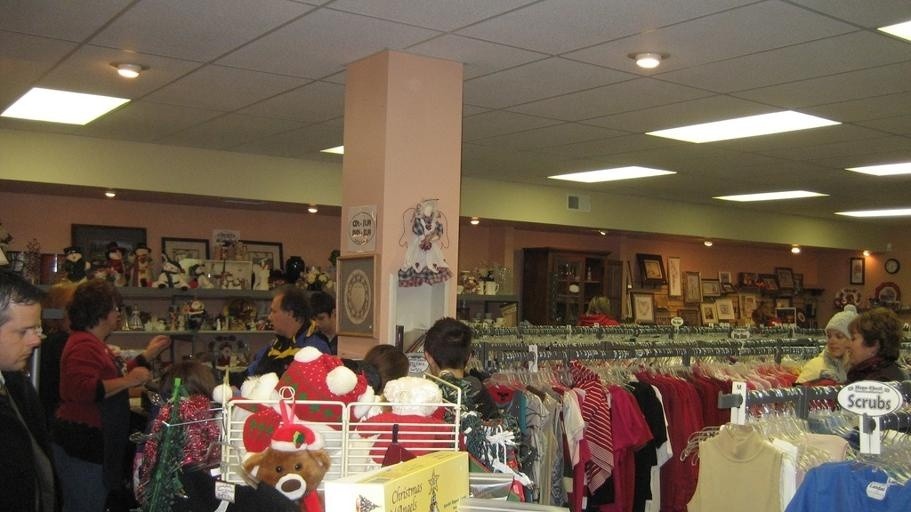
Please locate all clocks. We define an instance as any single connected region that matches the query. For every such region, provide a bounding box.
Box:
[885,258,900,273]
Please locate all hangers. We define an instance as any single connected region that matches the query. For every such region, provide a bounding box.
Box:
[680,385,860,472]
[848,413,911,487]
[465,326,911,389]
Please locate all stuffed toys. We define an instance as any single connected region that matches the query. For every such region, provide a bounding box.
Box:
[155,254,186,290]
[103,241,128,285]
[212,345,383,469]
[132,241,154,288]
[350,376,456,473]
[185,261,213,289]
[58,247,89,286]
[244,422,330,512]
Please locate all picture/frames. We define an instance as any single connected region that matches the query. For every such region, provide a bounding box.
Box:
[699,303,718,324]
[777,268,793,288]
[630,292,655,324]
[794,274,803,287]
[336,255,379,338]
[850,257,865,284]
[776,297,791,308]
[721,283,736,293]
[224,240,284,274]
[162,238,209,262]
[684,271,703,303]
[668,256,682,297]
[72,225,149,266]
[702,280,722,296]
[739,295,757,321]
[775,308,796,326]
[760,274,779,290]
[716,300,735,320]
[636,253,667,285]
[719,272,732,282]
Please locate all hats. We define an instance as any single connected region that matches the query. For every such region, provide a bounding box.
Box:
[824,304,860,339]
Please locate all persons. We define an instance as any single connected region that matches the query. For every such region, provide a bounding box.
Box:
[232,283,331,396]
[359,345,410,414]
[797,307,858,399]
[0,262,57,512]
[51,277,171,512]
[310,290,337,354]
[578,295,616,331]
[159,362,217,402]
[842,308,910,404]
[422,317,498,424]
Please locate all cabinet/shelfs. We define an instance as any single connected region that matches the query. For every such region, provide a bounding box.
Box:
[522,247,623,326]
[27,279,333,402]
[457,294,522,329]
